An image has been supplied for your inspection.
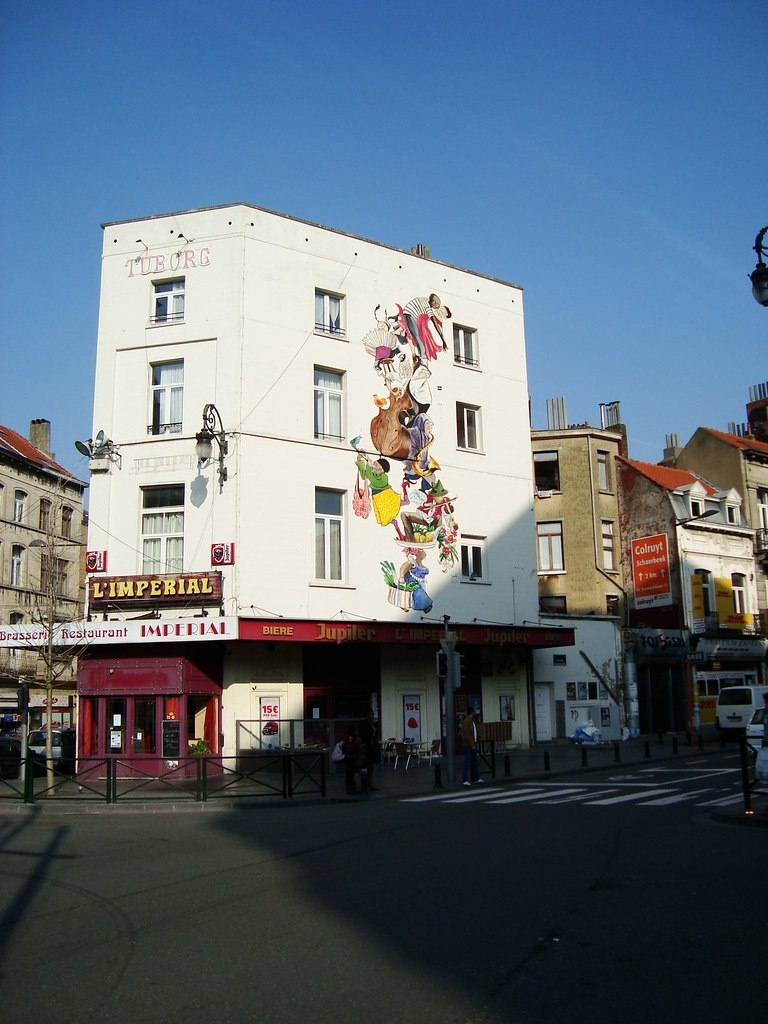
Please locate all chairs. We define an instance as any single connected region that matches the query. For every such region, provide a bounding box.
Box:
[378,738,441,770]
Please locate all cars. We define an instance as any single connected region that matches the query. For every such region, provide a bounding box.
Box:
[0,737,48,780]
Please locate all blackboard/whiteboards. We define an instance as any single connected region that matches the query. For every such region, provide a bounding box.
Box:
[161,719,180,759]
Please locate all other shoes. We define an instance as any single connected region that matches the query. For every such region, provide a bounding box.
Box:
[475,779,485,784]
[463,782,471,786]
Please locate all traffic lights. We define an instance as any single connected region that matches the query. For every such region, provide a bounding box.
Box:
[15,688,24,714]
[453,652,467,688]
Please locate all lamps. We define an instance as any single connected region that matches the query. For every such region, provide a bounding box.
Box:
[195,405,227,486]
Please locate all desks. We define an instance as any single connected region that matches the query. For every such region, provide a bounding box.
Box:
[404,742,426,765]
[378,741,403,765]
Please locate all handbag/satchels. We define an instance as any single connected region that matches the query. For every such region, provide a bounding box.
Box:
[755,746,768,780]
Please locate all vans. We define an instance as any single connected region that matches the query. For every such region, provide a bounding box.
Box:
[744,705,768,757]
[29,728,76,769]
[714,685,768,733]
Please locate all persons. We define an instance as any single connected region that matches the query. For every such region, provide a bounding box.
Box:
[460,706,486,786]
[343,710,382,797]
